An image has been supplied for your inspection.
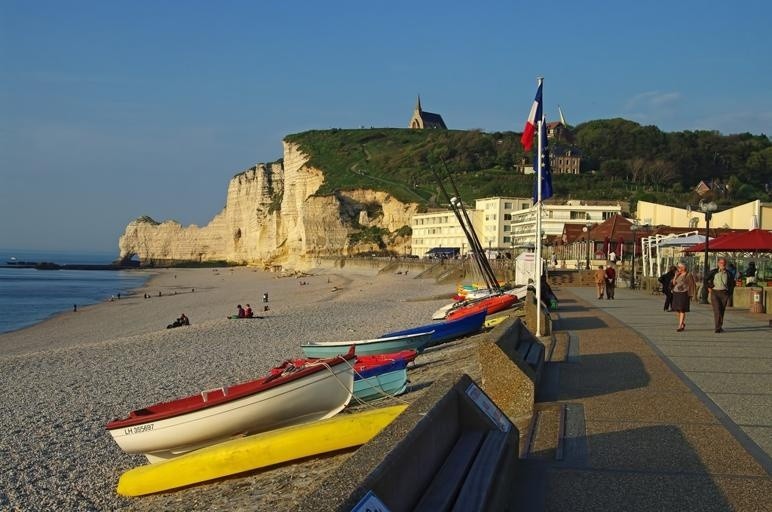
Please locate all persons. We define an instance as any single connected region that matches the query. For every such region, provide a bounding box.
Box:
[167,318,182,329]
[727,262,736,279]
[671,261,695,333]
[706,256,734,335]
[263,288,269,311]
[593,265,608,300]
[227,304,246,319]
[551,251,557,271]
[245,303,252,318]
[658,265,676,312]
[605,264,615,300]
[181,312,189,325]
[746,261,756,287]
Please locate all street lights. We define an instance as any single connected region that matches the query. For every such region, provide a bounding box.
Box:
[698,200,717,303]
[627,224,638,290]
[585,222,593,270]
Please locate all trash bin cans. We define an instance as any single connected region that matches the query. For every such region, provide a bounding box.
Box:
[751,289,765,314]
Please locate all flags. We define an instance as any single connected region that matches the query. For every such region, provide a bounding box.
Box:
[518,79,543,154]
[532,115,553,208]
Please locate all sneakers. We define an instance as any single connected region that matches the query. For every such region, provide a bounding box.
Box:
[715,328,723,333]
[677,324,685,332]
